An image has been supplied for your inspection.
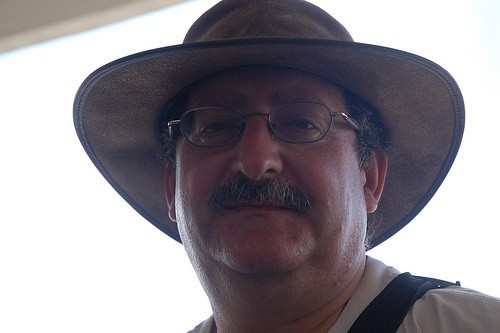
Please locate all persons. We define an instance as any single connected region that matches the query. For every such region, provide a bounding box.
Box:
[71,0,498,332]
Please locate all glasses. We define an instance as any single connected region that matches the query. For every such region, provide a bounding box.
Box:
[168,100,363,145]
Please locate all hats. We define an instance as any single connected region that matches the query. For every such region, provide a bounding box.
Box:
[73,0,465,251]
